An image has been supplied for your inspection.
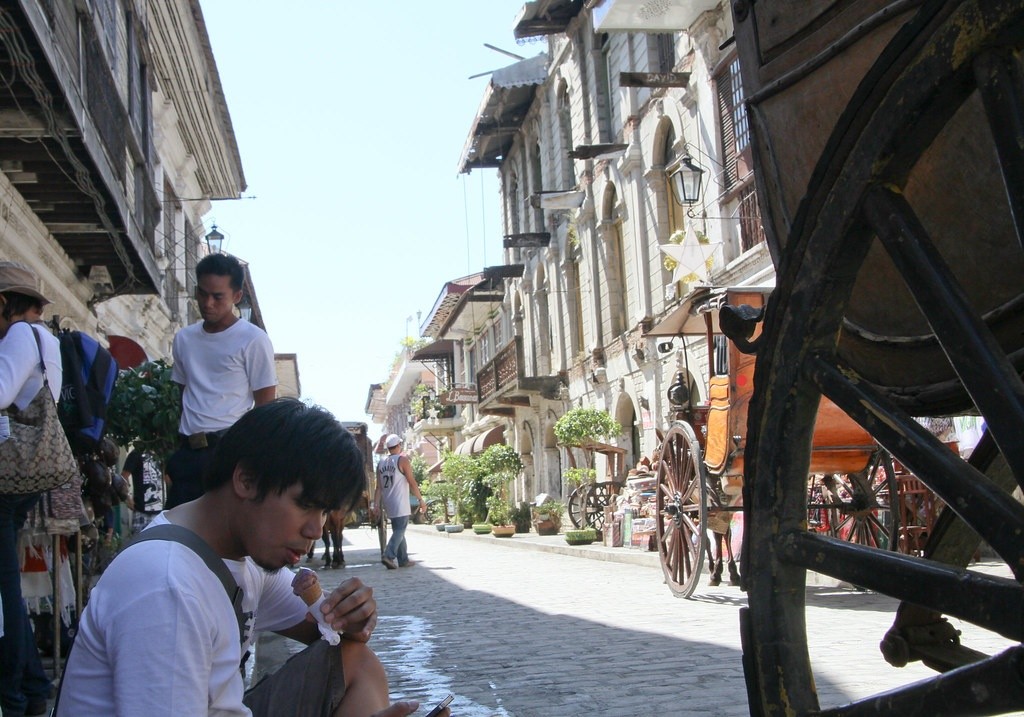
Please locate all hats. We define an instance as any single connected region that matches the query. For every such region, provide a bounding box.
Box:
[0,259,57,306]
[383,433,403,449]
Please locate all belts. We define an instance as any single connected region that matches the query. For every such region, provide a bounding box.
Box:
[177,428,231,451]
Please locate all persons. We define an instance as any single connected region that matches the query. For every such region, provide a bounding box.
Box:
[165,252,278,509]
[69,501,115,572]
[372,433,428,568]
[55,396,451,717]
[120,441,167,534]
[0,258,62,717]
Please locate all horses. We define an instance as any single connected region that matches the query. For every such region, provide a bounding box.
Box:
[655,428,739,584]
[322,503,345,569]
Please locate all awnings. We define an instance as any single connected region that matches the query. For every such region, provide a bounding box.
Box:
[513,0,586,44]
[457,54,550,174]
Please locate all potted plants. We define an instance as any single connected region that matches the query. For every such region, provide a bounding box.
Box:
[445,512,465,533]
[559,466,598,544]
[441,452,475,532]
[486,495,516,537]
[428,482,459,531]
[472,521,493,534]
[408,382,458,421]
[530,499,567,535]
[510,501,532,533]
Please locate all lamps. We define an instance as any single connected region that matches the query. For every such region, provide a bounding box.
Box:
[664,142,760,222]
[205,217,225,256]
[414,308,423,328]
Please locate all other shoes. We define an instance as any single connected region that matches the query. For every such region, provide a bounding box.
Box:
[0,693,48,717]
[381,556,397,569]
[399,560,416,567]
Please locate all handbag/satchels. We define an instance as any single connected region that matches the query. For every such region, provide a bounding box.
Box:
[0,319,78,497]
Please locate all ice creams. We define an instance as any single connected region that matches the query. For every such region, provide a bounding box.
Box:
[290,567,322,607]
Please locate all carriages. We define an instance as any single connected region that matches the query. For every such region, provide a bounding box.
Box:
[650,284,897,598]
[306,422,387,570]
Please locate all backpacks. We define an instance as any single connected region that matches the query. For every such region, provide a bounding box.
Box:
[45,313,121,455]
